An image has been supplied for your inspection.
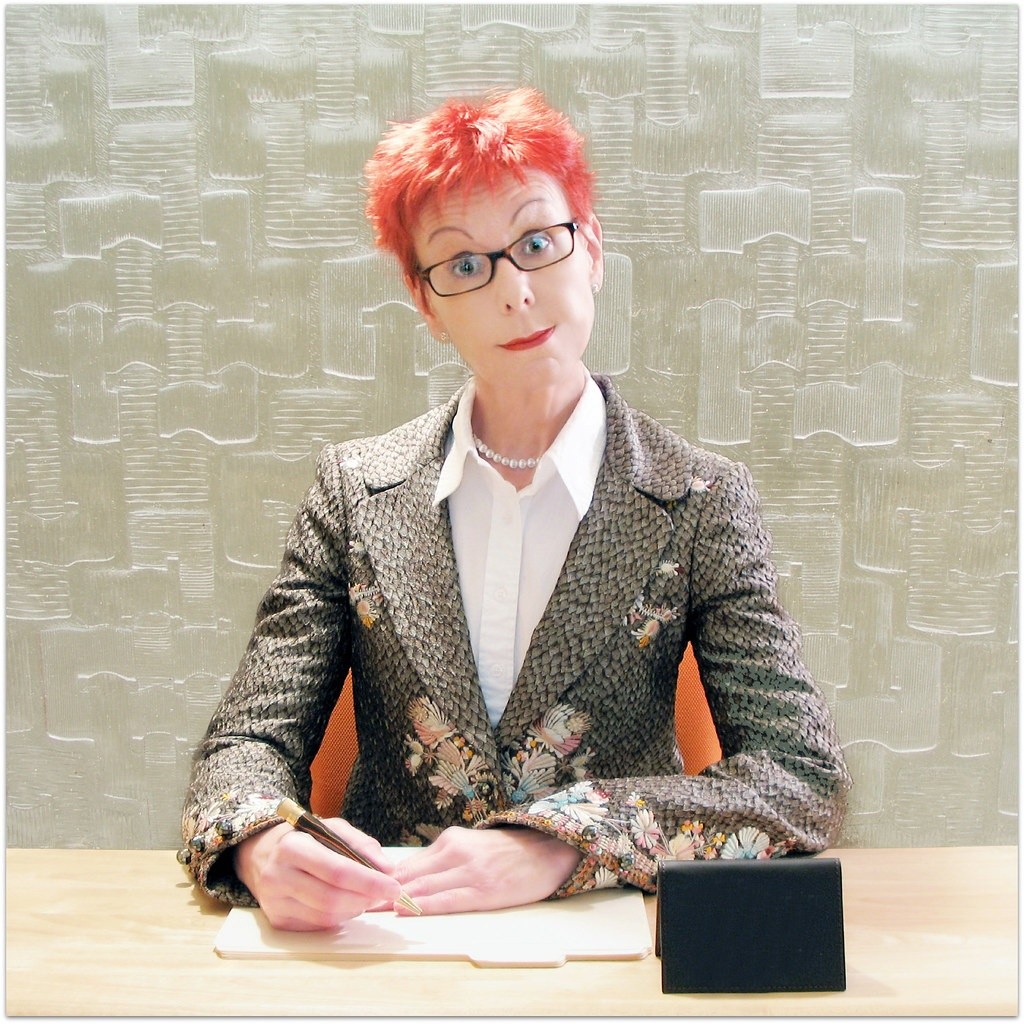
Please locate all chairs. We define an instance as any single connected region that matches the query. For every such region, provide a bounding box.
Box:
[310,638,724,821]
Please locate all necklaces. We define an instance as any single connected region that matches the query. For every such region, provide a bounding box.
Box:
[472,433,542,469]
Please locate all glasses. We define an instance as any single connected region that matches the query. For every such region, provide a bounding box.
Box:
[414,219,579,297]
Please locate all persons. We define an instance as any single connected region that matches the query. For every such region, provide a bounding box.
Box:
[176,88,852,932]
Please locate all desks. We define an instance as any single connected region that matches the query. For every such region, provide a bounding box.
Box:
[5,847,1019,1017]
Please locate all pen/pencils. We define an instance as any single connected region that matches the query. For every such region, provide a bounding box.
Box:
[272,798,422,916]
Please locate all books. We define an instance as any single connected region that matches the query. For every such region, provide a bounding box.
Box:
[213,846,653,969]
